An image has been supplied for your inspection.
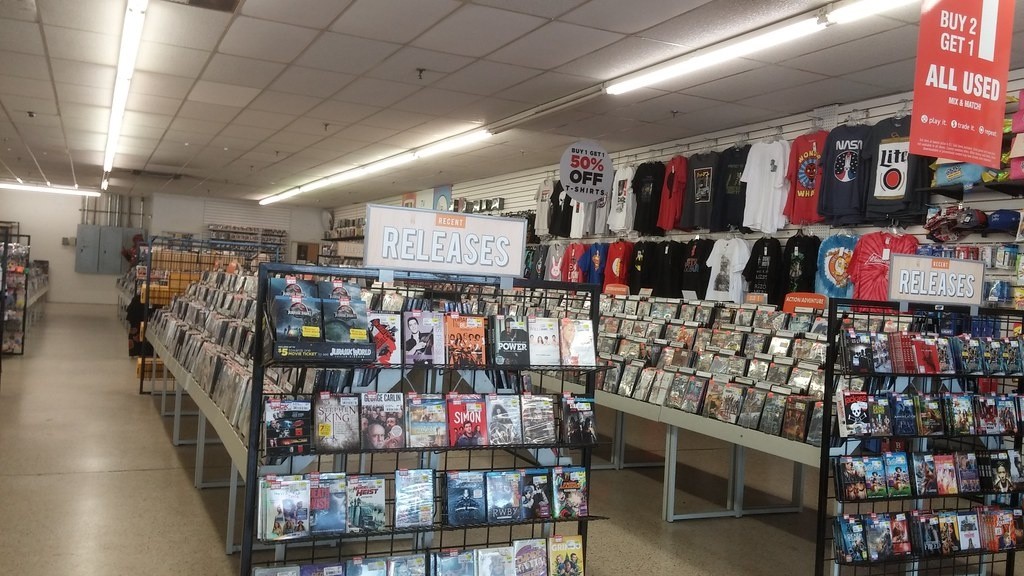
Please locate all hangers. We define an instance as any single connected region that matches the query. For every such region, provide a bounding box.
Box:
[501,210,537,252]
[539,214,906,249]
[543,100,911,182]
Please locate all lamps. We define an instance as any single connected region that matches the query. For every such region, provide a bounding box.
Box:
[101,0,147,192]
[258,83,606,206]
[604,0,923,97]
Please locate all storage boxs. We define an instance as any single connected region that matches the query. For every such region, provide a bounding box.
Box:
[472,197,503,212]
[935,158,991,187]
[1009,91,1024,180]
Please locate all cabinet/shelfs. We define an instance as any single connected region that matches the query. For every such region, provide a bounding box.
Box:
[142,262,990,576]
[208,228,364,266]
[5,268,48,331]
[114,267,144,333]
[915,101,1024,202]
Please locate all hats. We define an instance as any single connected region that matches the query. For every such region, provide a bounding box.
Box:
[951,209,988,231]
[923,205,969,242]
[982,209,1020,234]
